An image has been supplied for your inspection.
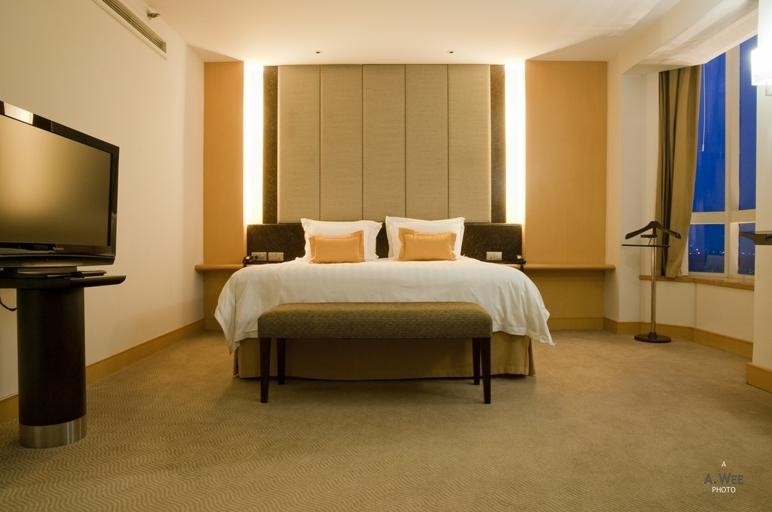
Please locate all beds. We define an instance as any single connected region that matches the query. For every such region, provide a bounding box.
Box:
[235,221,531,379]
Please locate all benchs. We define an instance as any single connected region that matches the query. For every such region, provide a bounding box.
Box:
[258,301,492,404]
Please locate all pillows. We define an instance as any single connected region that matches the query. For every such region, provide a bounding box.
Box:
[293,215,466,264]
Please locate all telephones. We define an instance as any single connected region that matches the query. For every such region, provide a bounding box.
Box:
[243,256,254,263]
[511,254,526,264]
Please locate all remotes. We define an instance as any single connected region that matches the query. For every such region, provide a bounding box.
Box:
[75,268,107,276]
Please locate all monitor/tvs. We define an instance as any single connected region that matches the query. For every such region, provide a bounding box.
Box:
[0,101,119,276]
